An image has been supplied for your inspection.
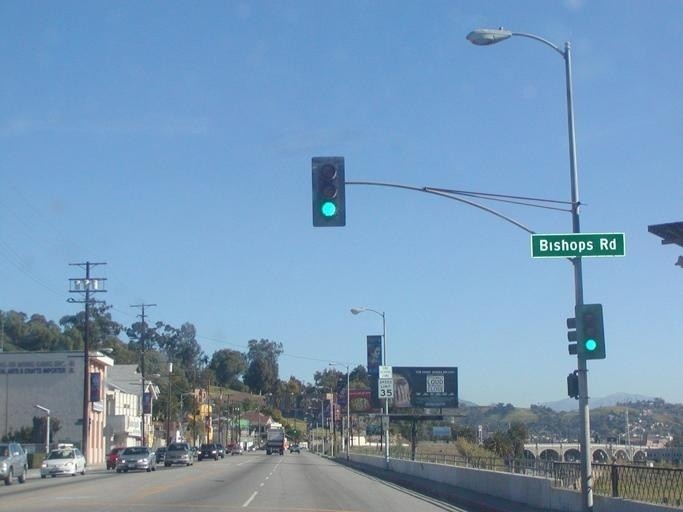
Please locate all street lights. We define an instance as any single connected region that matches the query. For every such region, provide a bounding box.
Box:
[351,306,389,469]
[466,27,594,509]
[33,403,50,458]
[329,360,350,460]
[304,397,325,456]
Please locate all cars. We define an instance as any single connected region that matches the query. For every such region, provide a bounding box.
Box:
[40,444,87,477]
[0,442,28,485]
[198,442,225,461]
[105,446,157,472]
[164,442,193,466]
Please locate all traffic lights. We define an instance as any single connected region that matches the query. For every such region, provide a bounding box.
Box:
[312,157,345,227]
[566,304,607,360]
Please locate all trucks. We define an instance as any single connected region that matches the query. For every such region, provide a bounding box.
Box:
[266,426,284,455]
